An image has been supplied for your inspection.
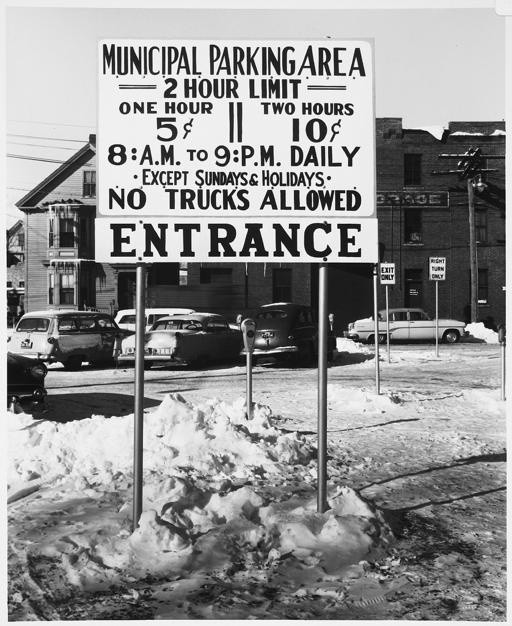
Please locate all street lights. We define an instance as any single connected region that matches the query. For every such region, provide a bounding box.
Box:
[464,171,490,322]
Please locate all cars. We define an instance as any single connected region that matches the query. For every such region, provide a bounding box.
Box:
[116,308,248,371]
[240,299,340,366]
[343,306,469,344]
[7,347,54,420]
[6,306,136,370]
[90,302,242,328]
